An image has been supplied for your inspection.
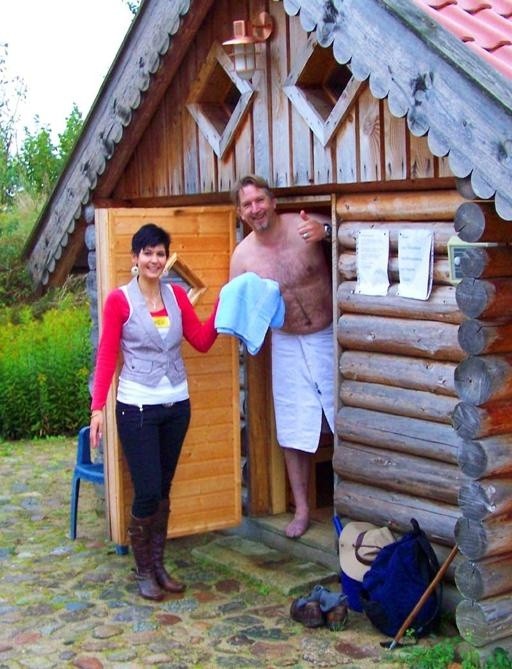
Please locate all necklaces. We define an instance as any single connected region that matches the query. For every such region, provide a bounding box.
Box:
[140,285,162,309]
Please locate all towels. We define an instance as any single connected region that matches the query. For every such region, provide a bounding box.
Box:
[213,271,288,358]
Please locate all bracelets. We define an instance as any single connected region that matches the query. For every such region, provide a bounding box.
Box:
[91,412,100,418]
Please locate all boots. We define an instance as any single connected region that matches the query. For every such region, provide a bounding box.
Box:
[128,513,165,601]
[151,500,187,593]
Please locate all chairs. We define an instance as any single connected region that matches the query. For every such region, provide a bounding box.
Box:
[69,426,130,557]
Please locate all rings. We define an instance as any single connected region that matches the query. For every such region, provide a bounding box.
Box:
[303,233,308,239]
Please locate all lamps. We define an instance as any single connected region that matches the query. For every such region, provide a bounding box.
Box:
[224,11,276,80]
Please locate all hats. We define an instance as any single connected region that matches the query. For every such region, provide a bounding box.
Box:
[337,520,394,582]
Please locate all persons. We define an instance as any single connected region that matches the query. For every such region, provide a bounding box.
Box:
[228,176,336,539]
[90,223,219,601]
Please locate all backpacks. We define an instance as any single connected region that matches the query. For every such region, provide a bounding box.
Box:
[361,518,444,650]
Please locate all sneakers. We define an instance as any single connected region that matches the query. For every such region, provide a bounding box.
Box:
[326,602,348,631]
[290,595,323,628]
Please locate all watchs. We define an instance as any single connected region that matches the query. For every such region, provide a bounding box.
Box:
[323,223,332,236]
